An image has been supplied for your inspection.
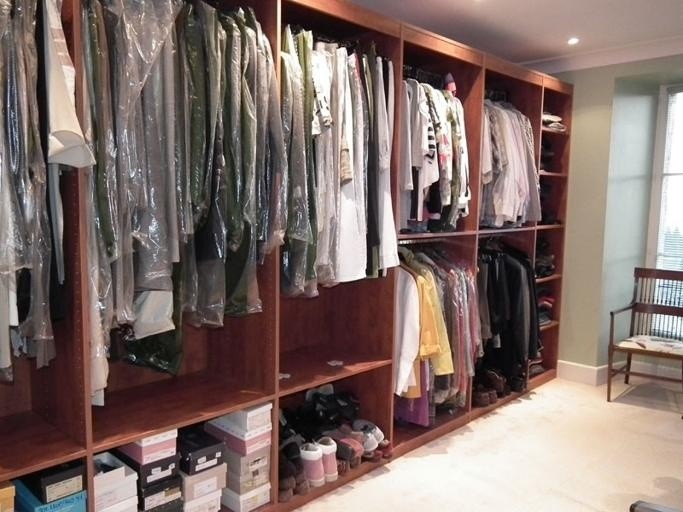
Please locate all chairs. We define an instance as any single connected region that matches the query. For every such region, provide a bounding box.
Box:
[605,264,683,402]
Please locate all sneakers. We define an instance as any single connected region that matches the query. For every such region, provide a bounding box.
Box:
[279,380,394,503]
[472,338,546,408]
